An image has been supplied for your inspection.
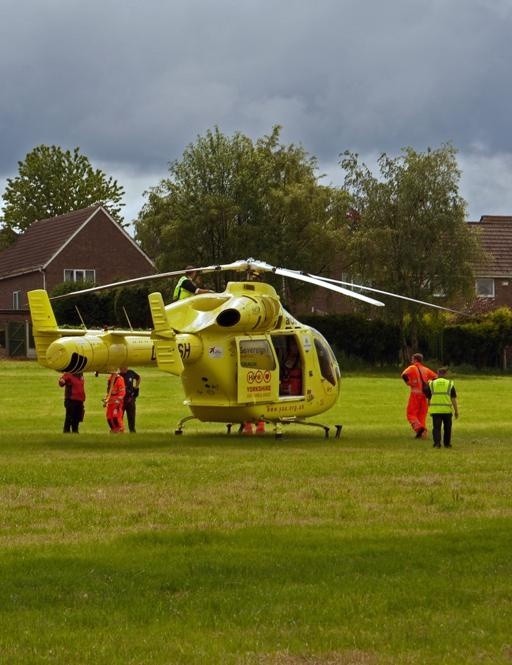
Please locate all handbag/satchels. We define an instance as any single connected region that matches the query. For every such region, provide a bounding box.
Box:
[415,364,432,399]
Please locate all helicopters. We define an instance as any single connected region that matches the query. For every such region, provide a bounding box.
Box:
[22,255,482,440]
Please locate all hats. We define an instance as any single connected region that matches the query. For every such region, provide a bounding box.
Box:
[438,365,450,373]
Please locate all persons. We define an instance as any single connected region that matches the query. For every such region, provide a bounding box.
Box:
[172,265,215,304]
[400,353,439,439]
[103,373,126,432]
[427,368,459,449]
[58,371,85,433]
[118,366,141,433]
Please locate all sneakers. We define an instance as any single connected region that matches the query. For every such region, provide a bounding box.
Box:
[414,427,428,441]
